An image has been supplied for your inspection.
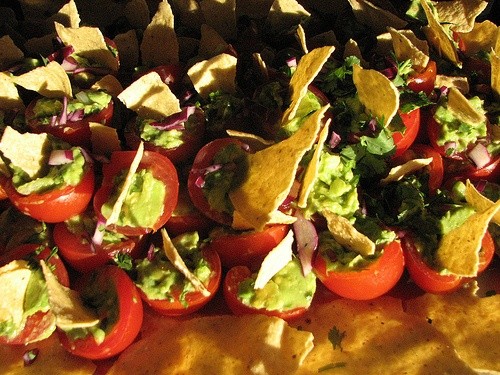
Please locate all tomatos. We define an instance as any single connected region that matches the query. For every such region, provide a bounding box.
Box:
[0,75,500,359]
